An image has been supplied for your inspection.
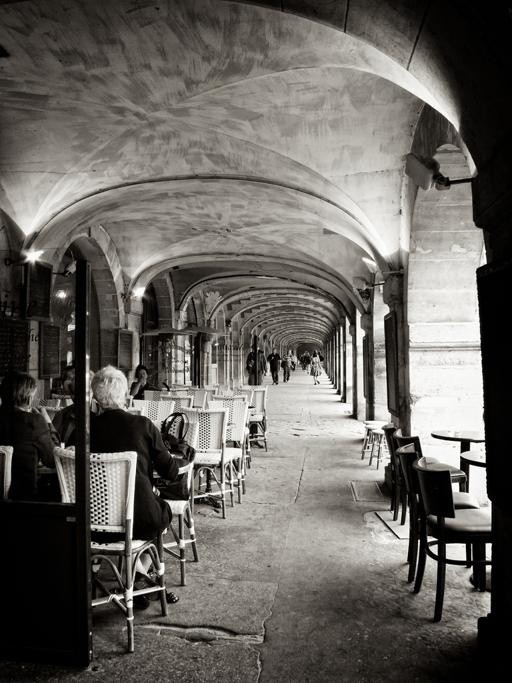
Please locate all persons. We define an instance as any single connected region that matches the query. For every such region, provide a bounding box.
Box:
[0,371,59,500]
[244,346,324,386]
[52,367,104,447]
[65,364,178,610]
[129,363,163,401]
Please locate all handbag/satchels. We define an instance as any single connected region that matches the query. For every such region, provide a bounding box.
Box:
[153,432,196,501]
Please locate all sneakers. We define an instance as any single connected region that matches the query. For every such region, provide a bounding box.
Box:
[133,572,160,610]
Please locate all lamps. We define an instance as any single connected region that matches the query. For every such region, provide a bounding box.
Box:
[402,149,474,194]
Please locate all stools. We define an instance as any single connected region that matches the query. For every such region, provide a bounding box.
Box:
[360,420,391,470]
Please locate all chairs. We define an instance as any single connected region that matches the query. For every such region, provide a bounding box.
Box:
[382,420,493,623]
